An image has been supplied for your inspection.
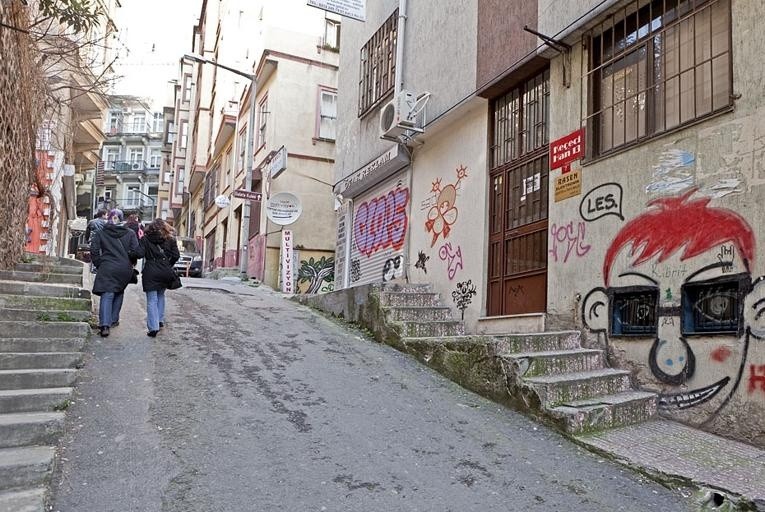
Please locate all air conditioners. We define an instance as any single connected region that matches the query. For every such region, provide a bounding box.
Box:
[378,90,416,141]
[132,162,144,171]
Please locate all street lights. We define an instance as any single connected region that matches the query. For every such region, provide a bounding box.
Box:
[183,51,257,278]
[132,187,155,222]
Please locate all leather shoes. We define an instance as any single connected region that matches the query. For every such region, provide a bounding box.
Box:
[100,321,119,337]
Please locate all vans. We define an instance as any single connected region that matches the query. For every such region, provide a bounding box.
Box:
[172,235,203,279]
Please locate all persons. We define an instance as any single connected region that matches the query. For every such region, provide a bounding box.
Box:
[85,209,182,337]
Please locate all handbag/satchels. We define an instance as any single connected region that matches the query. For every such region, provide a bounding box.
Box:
[167,270,182,290]
[128,267,139,284]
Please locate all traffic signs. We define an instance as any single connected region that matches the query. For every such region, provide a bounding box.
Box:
[233,189,263,203]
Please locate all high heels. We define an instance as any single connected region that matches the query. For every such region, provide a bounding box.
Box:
[148,321,164,337]
